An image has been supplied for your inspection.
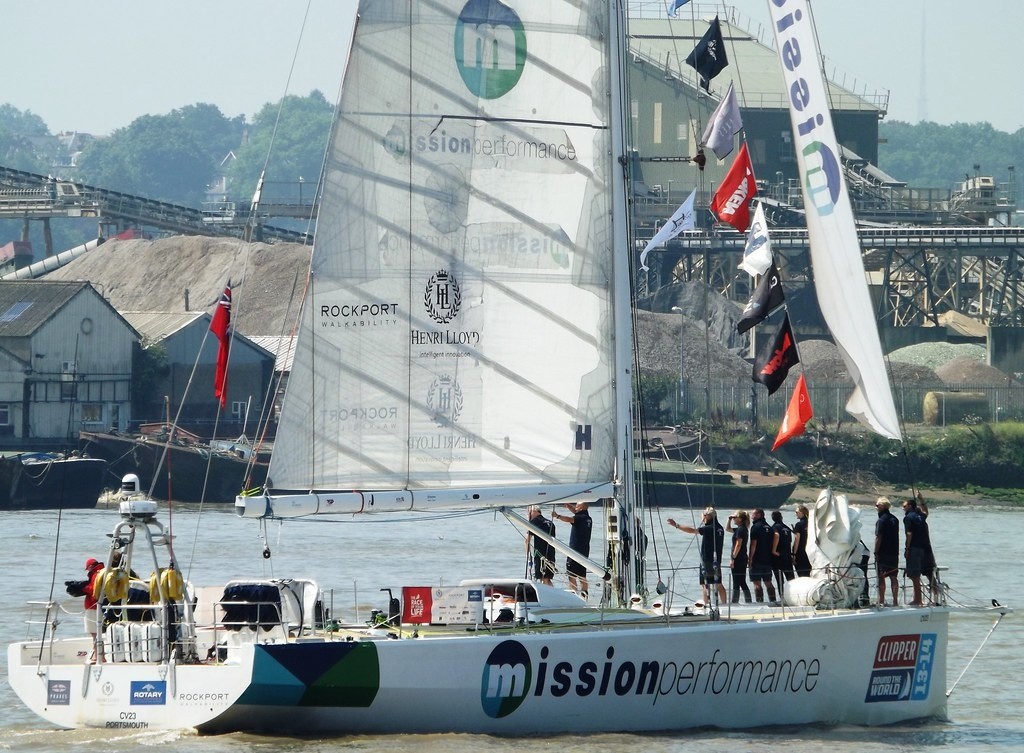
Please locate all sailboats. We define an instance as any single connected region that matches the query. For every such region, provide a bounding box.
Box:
[8,0,952,731]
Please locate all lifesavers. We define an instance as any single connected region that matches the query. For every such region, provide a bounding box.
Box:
[92,566,130,603]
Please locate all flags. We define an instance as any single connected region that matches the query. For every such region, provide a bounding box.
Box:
[208,277,232,412]
[684,15,729,83]
[698,81,747,161]
[666,0,690,17]
[769,373,815,453]
[736,199,775,278]
[750,311,800,397]
[735,256,787,335]
[709,141,759,235]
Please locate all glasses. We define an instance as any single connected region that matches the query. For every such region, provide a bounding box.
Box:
[875,504,881,507]
[732,516,740,519]
[796,511,799,513]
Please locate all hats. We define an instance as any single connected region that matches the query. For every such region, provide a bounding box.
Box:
[85,558,96,569]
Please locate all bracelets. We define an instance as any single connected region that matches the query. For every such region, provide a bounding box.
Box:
[874,552,879,555]
[675,522,679,529]
[905,548,912,550]
[557,515,560,519]
[793,554,797,556]
[731,556,736,561]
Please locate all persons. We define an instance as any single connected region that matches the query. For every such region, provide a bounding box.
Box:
[634,517,648,594]
[873,496,900,609]
[901,491,942,607]
[725,510,752,604]
[666,506,727,609]
[551,501,593,600]
[748,507,777,603]
[790,505,812,577]
[770,510,796,607]
[79,557,107,664]
[524,505,556,588]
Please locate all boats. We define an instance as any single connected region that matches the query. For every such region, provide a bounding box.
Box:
[80,426,798,509]
